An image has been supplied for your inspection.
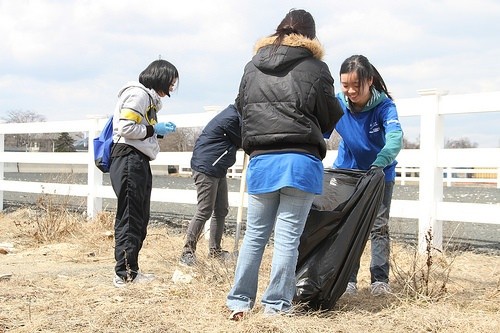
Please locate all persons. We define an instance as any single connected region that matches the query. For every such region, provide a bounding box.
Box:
[110,59,178,287]
[226,10,343,318]
[321,55,405,296]
[178,102,242,266]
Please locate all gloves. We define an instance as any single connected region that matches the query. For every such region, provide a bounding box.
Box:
[154,122,177,135]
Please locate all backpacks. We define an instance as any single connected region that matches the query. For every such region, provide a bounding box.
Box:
[93,97,151,173]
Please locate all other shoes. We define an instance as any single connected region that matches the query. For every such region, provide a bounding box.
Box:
[208,251,226,260]
[226,310,244,321]
[345,281,358,295]
[181,255,197,267]
[371,281,390,297]
[114,272,155,288]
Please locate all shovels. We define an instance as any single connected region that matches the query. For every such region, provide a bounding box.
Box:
[222,154,250,273]
